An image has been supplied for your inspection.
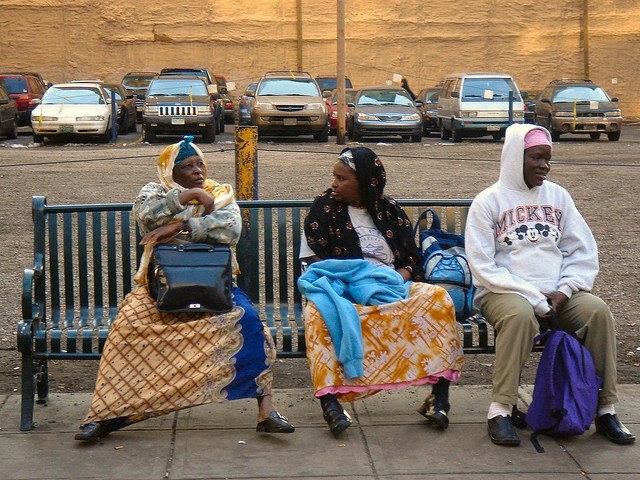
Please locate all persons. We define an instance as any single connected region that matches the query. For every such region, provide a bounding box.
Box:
[73,135,295,441]
[297,147,464,438]
[464,122,636,447]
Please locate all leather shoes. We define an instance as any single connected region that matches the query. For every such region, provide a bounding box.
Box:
[75,422,111,440]
[597,412,638,445]
[487,414,520,445]
[256,410,296,434]
[322,401,353,436]
[418,394,450,428]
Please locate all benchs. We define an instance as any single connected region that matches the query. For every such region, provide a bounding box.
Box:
[18,196,554,432]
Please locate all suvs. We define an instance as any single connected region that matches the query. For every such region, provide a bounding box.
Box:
[314,75,353,101]
[245,70,331,143]
[137,76,221,142]
[239,82,258,126]
[121,72,160,121]
[213,75,227,93]
[534,78,622,141]
[161,67,225,135]
[0,72,46,127]
[437,72,525,142]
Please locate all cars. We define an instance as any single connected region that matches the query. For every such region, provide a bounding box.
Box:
[219,94,234,124]
[522,97,536,121]
[100,83,138,135]
[414,87,443,137]
[346,86,424,142]
[30,83,118,143]
[0,85,20,139]
[325,88,360,136]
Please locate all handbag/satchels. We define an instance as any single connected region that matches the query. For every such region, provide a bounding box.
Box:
[148,244,233,314]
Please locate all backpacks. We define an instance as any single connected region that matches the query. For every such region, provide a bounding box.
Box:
[525,328,601,454]
[413,209,475,318]
